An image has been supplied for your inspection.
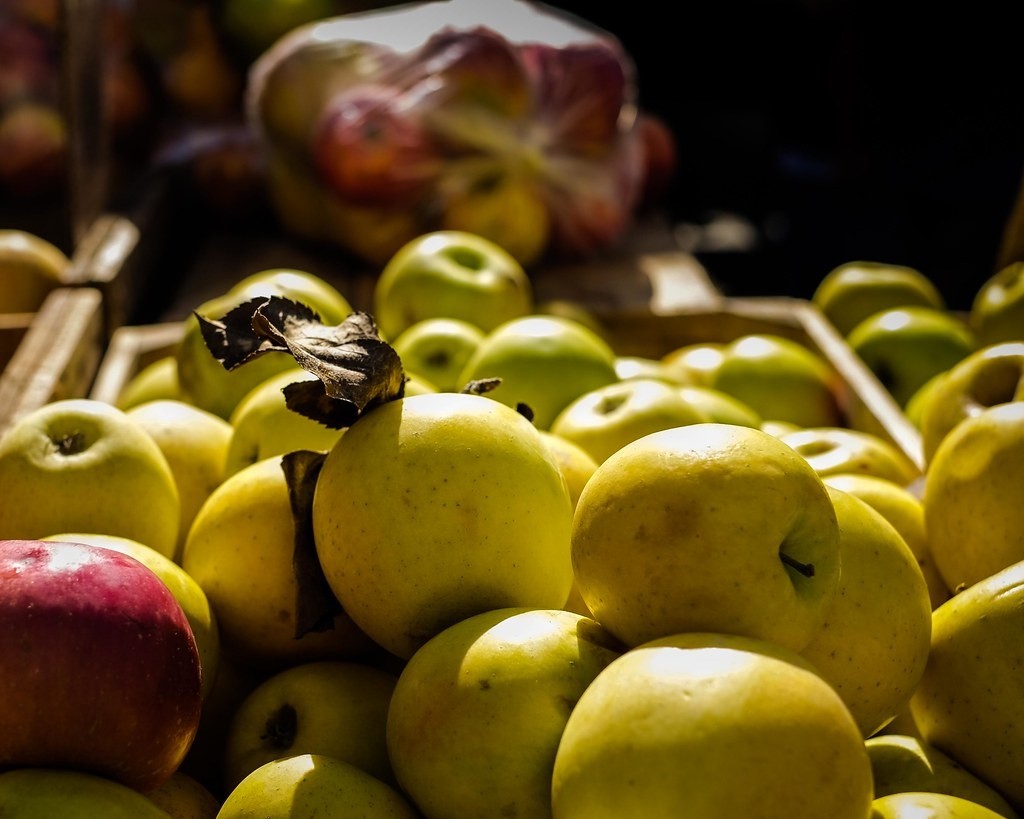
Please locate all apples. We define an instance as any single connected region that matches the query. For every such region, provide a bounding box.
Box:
[0,0,1024,819]
